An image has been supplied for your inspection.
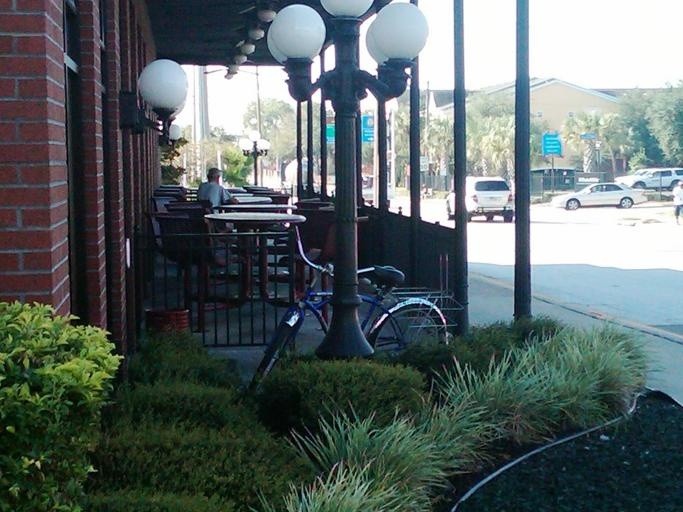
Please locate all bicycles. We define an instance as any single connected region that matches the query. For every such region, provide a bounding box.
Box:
[248,220,466,396]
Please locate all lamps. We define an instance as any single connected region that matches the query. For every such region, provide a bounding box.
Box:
[239,0,276,57]
[119,61,189,142]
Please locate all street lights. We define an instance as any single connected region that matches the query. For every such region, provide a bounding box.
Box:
[266,2,430,362]
[240,129,272,186]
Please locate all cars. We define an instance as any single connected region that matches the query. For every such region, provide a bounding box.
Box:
[614,167,683,191]
[550,182,648,211]
[444,174,514,222]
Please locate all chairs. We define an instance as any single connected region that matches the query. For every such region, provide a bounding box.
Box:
[144,185,338,333]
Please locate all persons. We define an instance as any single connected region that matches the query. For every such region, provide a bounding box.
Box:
[196,168,240,215]
[672,182,683,224]
